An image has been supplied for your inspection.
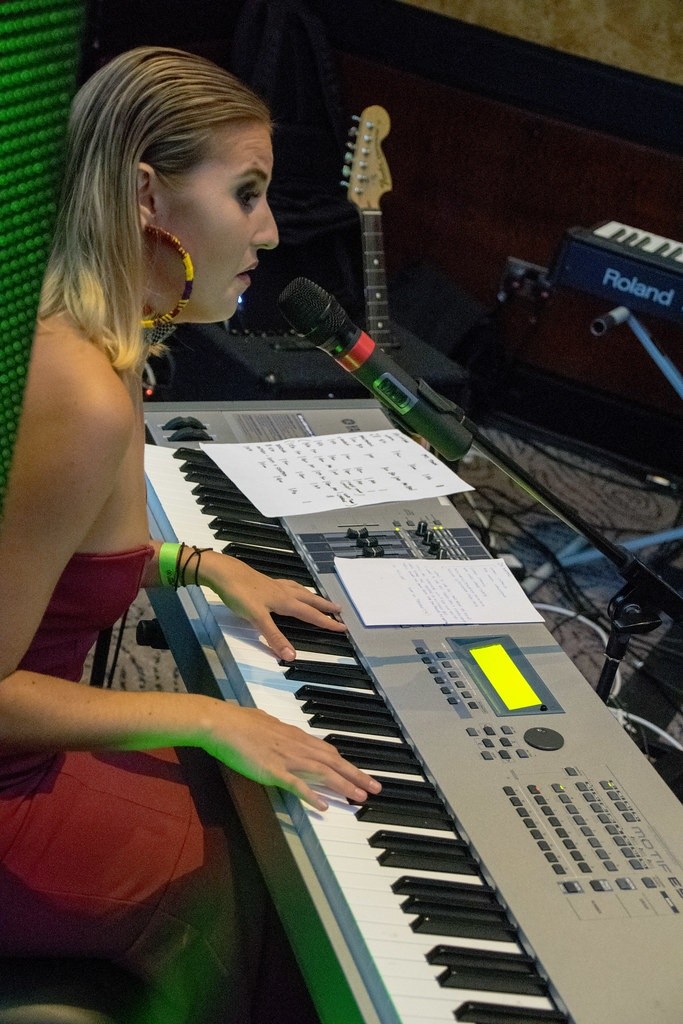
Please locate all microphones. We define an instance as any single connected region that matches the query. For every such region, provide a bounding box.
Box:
[275,277,473,461]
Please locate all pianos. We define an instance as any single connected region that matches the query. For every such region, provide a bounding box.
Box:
[550,218,683,313]
[134,398,682,1024]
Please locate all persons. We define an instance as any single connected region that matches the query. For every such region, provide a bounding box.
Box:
[0,46,382,1024]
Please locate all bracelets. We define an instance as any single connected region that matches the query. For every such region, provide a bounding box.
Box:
[159,542,213,591]
